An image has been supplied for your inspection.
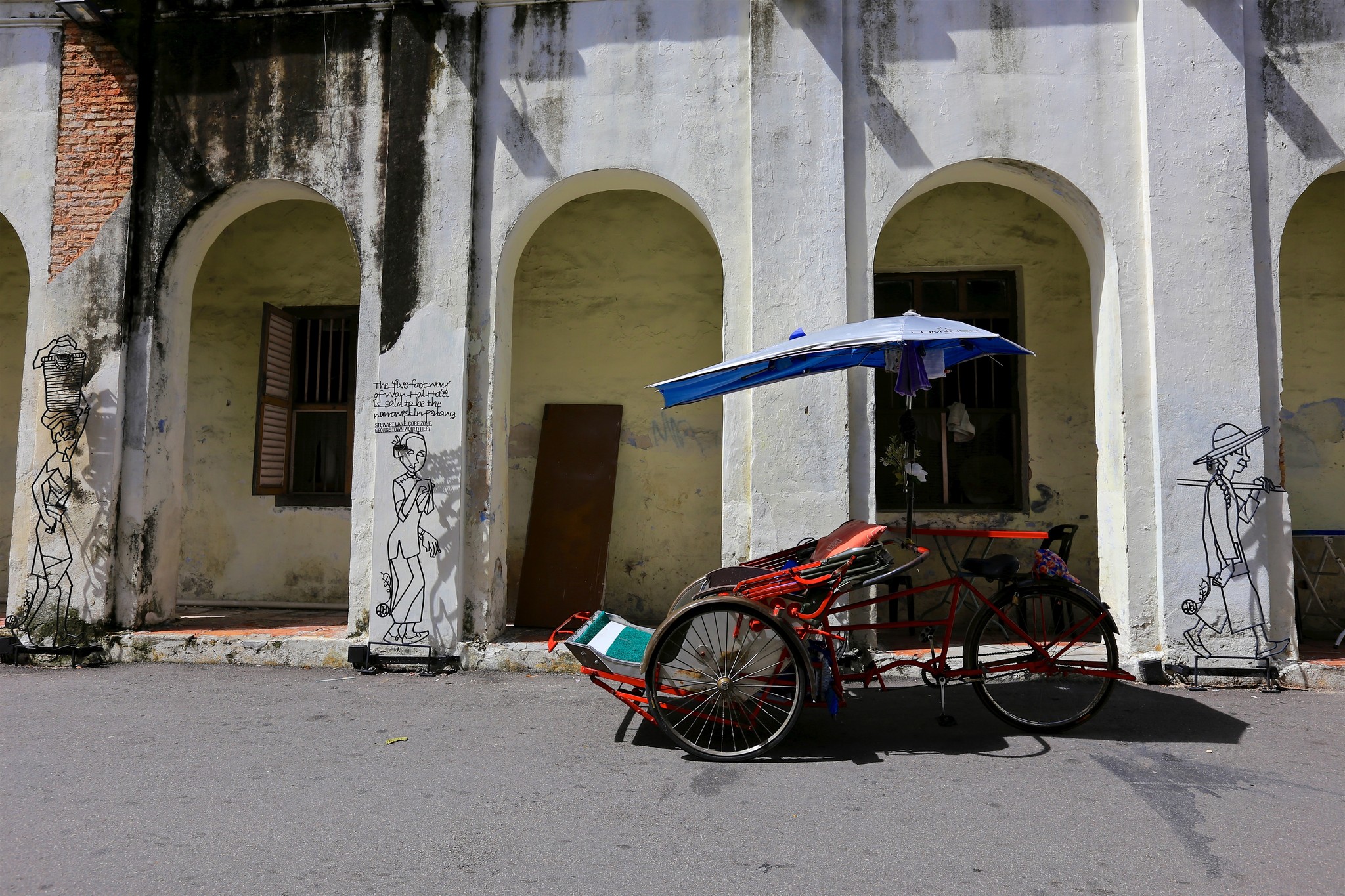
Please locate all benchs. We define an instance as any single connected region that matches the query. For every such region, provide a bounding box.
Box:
[692,516,888,613]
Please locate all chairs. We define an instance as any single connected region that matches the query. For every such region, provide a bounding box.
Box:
[996,523,1080,638]
[875,573,917,639]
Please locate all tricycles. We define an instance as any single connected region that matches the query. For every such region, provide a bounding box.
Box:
[548,310,1136,762]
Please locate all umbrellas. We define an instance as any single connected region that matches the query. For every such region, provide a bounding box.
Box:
[649,309,1036,537]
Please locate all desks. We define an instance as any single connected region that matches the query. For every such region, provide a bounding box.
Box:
[887,526,1049,642]
[1291,529,1345,650]
